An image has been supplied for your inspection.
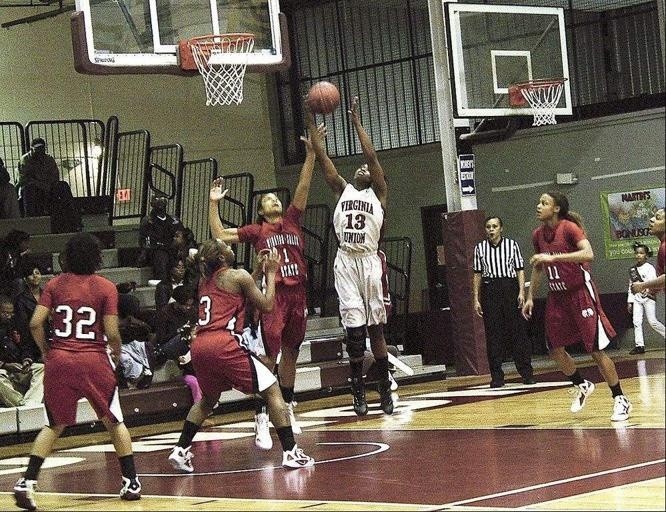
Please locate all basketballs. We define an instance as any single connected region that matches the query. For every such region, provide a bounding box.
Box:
[309,81,339,113]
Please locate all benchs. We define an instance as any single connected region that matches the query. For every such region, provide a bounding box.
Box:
[0,211,446,434]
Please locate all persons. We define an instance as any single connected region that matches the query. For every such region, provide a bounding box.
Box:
[521,191,632,422]
[1,229,54,408]
[115,195,202,404]
[237,263,282,365]
[208,132,316,451]
[472,215,536,389]
[628,244,666,355]
[630,206,665,296]
[166,239,317,470]
[310,95,392,416]
[367,249,398,392]
[1,139,78,217]
[12,233,140,511]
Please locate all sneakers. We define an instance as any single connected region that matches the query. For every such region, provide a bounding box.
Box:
[119,474,143,501]
[168,370,398,473]
[610,395,634,421]
[12,477,40,509]
[569,380,595,413]
[629,347,645,355]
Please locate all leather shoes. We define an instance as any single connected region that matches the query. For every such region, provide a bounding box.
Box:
[490,379,504,388]
[522,377,538,385]
